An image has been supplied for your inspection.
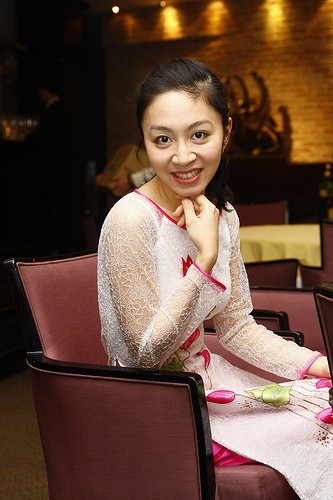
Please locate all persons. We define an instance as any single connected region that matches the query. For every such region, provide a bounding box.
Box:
[97,59,333,500]
[22,76,88,254]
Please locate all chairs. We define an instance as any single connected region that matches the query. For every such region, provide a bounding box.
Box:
[234,156,333,381]
[3,249,305,500]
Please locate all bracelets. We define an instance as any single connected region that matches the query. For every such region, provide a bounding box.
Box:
[127,174,136,192]
